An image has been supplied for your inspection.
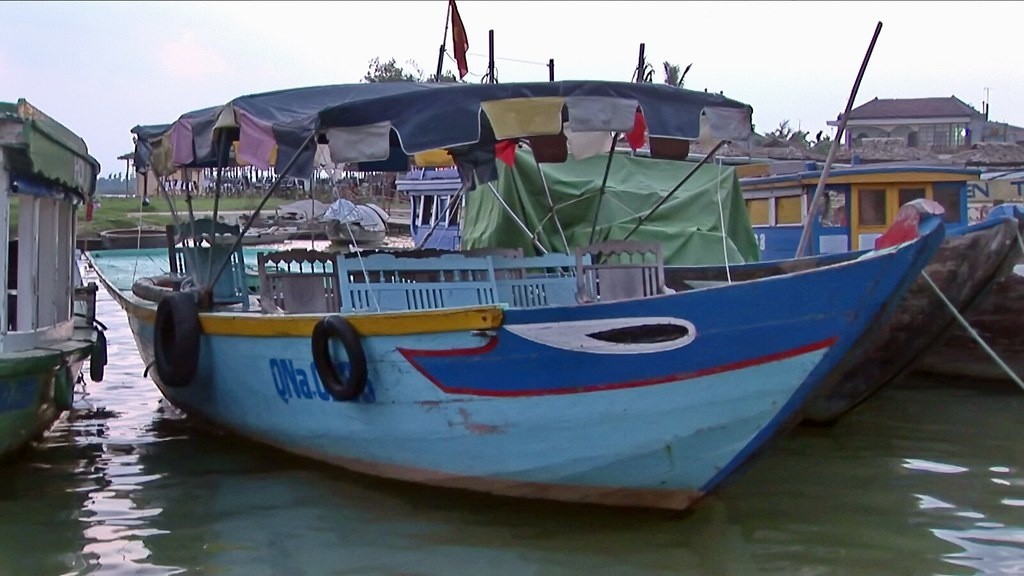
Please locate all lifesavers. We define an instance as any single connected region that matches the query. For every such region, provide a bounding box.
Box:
[153,289,201,390]
[311,315,368,401]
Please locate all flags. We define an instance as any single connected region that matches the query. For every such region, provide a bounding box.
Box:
[451,0,469,81]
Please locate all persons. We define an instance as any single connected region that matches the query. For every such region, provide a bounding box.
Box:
[816,192,848,228]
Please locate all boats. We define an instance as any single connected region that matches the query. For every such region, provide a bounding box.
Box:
[83,6,1024,526]
[0,97,108,473]
